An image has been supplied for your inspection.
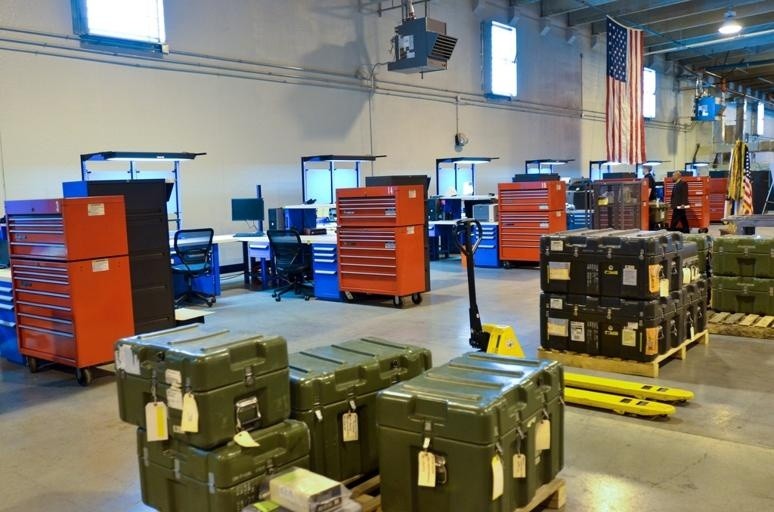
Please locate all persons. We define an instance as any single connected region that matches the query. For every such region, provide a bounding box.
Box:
[641,162,656,202]
[669,170,693,233]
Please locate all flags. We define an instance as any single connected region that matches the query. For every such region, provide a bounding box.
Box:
[741,150,754,216]
[606,16,646,165]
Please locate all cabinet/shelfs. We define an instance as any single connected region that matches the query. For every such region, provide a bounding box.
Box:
[564,209,595,229]
[0,267,24,368]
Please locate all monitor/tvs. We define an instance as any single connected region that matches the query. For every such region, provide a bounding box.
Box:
[231,198,264,221]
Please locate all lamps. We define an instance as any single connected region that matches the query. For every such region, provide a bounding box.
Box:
[715,0,742,34]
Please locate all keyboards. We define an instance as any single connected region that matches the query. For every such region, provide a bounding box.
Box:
[232,232,264,237]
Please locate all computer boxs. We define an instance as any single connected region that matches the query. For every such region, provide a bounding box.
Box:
[268,208,284,234]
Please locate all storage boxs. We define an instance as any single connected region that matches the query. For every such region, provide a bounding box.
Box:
[712,274,774,316]
[712,233,774,279]
[537,228,711,364]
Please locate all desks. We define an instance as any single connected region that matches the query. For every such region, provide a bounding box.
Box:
[428,219,499,269]
[169,229,341,302]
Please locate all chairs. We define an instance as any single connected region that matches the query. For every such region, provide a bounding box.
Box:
[267,228,311,301]
[171,226,217,308]
